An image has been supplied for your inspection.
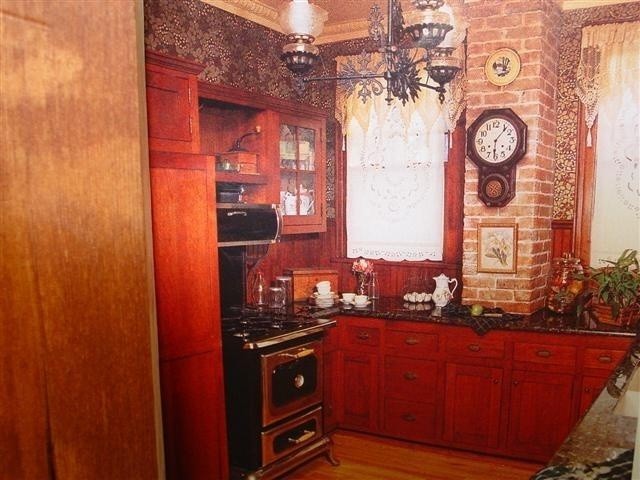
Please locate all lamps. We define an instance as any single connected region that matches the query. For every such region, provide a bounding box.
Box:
[270,0,468,104]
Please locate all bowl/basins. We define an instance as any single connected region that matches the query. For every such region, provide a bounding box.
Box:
[218,185,244,202]
[216,139,258,174]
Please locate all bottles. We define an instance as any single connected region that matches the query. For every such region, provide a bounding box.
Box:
[280,139,314,171]
[372,272,379,301]
[545,251,585,314]
[254,271,292,308]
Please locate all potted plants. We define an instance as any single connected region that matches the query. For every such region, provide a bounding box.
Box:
[576,248,639,329]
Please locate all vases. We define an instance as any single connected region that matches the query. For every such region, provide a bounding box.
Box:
[357,274,368,296]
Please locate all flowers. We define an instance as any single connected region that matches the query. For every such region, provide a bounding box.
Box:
[352,257,375,277]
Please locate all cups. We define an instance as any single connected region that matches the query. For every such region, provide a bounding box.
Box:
[312,281,371,308]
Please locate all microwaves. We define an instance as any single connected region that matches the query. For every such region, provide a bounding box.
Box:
[218,200,281,249]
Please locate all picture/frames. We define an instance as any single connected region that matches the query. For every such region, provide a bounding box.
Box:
[485,48,522,86]
[476,220,519,275]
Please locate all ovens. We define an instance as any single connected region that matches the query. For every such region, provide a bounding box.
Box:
[227,337,340,480]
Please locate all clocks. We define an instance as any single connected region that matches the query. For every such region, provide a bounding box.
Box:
[462,107,530,207]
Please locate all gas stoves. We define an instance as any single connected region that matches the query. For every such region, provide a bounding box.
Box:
[223,305,335,353]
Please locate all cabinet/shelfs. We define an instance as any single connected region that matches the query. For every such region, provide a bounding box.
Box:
[580,333,637,419]
[384,321,443,444]
[443,324,580,465]
[144,149,232,480]
[331,317,383,435]
[197,79,271,207]
[144,48,208,155]
[275,112,330,235]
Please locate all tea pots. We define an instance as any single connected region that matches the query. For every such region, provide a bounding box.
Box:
[282,183,315,216]
[433,274,457,307]
